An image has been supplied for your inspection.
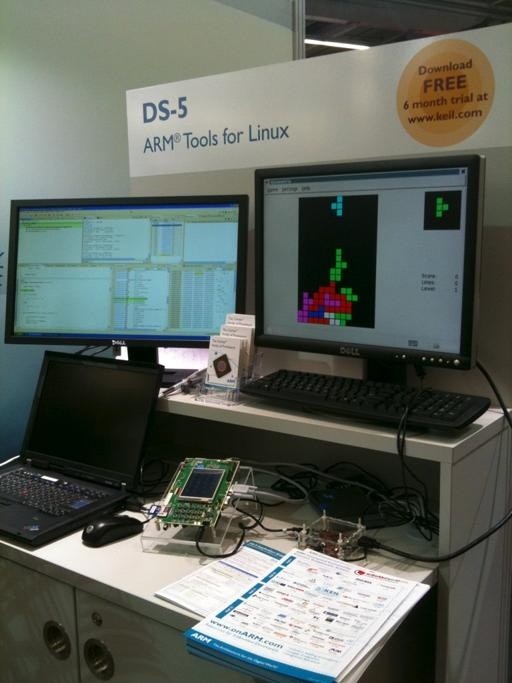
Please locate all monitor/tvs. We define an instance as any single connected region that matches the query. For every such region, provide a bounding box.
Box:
[253,153,487,390]
[4,194,250,390]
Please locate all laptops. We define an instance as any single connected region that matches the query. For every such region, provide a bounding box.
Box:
[0,350,166,551]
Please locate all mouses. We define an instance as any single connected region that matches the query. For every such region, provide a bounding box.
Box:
[81,515,143,547]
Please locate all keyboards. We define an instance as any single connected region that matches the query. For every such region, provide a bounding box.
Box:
[239,367,492,435]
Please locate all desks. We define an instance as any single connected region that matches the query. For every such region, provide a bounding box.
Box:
[0,452,440,683]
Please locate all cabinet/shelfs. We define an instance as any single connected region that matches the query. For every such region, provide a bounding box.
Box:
[157,381,511,681]
